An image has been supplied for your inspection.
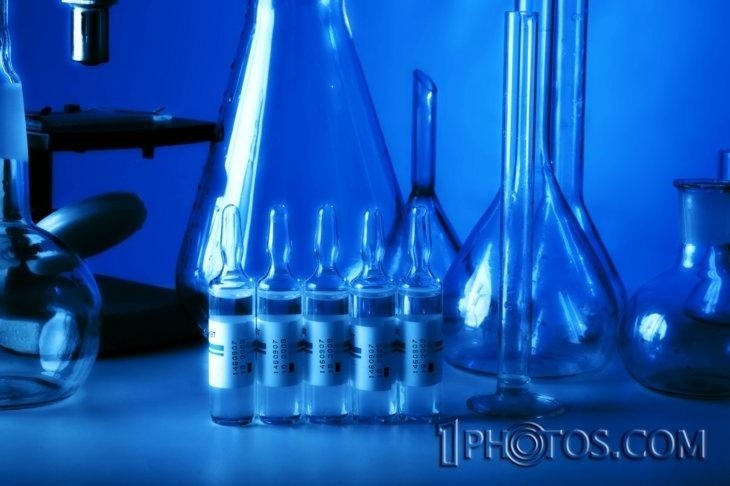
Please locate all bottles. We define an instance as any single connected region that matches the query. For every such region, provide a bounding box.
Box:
[0,0,729,428]
